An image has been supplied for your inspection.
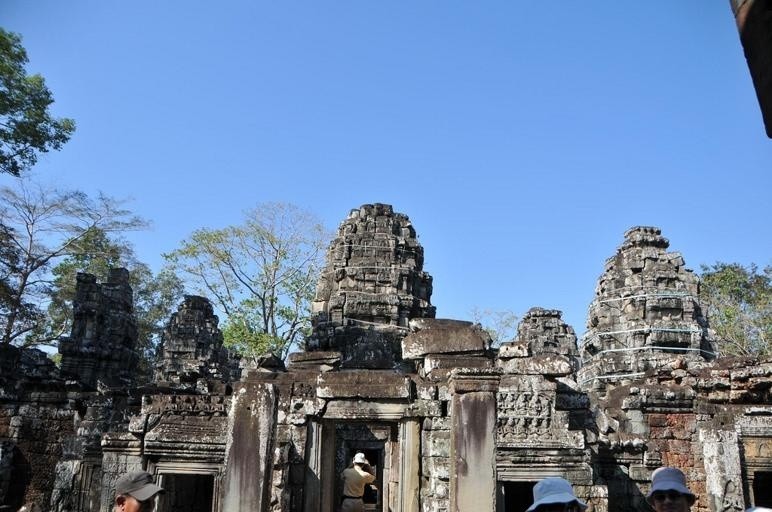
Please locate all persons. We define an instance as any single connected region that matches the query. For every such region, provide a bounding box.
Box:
[0,440,30,512]
[338,453,376,512]
[112,469,165,512]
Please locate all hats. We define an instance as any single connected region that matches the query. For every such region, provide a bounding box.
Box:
[353,453,365,464]
[116,472,166,501]
